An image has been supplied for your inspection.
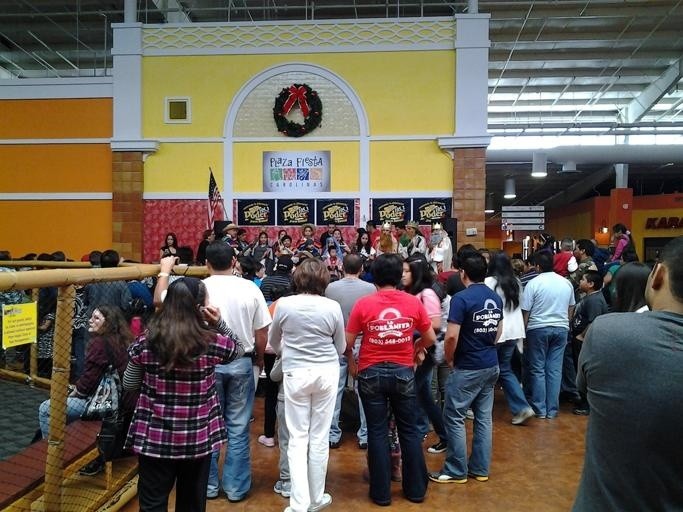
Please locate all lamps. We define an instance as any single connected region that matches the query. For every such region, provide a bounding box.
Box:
[484,192,495,216]
[599,219,609,234]
[531,151,549,179]
[502,179,518,200]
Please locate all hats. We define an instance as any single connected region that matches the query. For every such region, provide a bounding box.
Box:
[220,224,238,233]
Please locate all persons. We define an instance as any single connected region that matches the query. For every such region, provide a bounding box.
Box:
[122,221,453,512]
[39,305,135,474]
[427,222,653,483]
[0,249,159,396]
[577,233,683,512]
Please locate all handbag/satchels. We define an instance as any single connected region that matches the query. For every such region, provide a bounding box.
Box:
[80,336,134,458]
[338,365,360,433]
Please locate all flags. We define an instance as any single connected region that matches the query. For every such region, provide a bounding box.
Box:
[205,172,221,230]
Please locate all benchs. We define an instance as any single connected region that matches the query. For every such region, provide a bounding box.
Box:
[1,418,115,512]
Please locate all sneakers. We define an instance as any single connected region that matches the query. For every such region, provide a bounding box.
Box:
[359,438,488,506]
[273,480,332,512]
[79,461,104,476]
[511,408,553,424]
[257,435,275,447]
